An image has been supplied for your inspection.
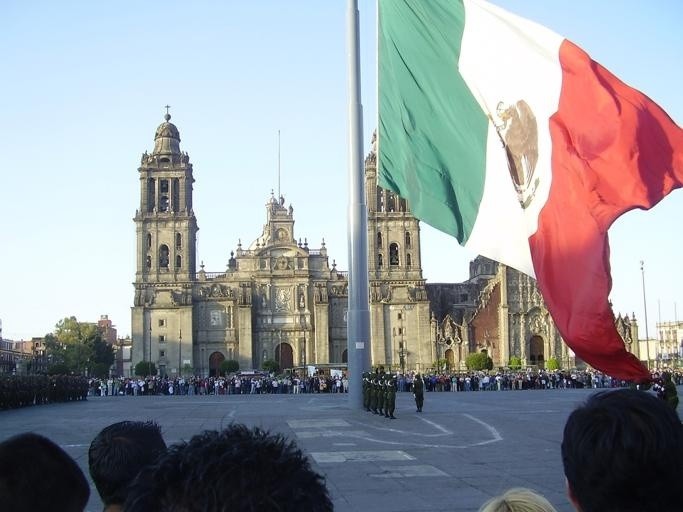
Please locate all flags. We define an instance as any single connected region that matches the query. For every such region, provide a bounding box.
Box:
[377,0,683,384]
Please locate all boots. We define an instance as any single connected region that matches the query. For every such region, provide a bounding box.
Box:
[366,407,397,419]
[416,407,422,412]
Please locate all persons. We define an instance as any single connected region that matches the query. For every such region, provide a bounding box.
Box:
[88,419,167,512]
[1,365,683,422]
[477,485,558,512]
[0,430,91,512]
[558,388,683,512]
[121,421,335,512]
